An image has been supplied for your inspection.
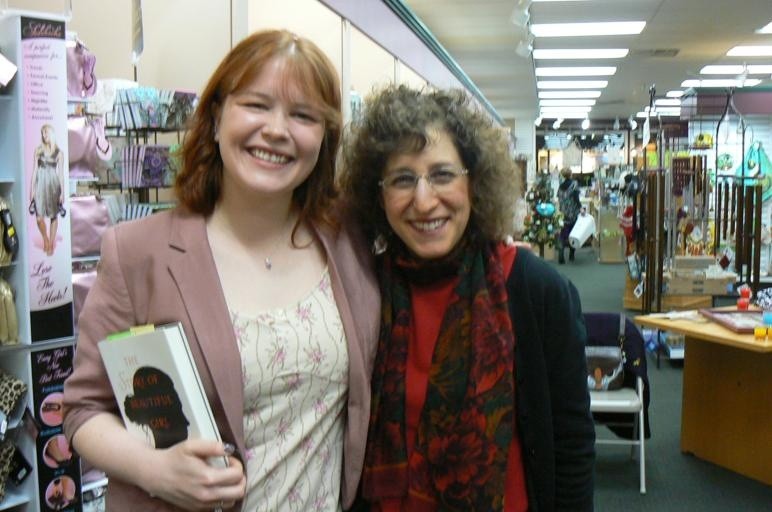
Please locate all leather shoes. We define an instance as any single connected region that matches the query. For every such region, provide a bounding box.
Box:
[29,200,66,218]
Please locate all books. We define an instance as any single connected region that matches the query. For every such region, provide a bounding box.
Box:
[87,83,195,225]
[95,319,234,499]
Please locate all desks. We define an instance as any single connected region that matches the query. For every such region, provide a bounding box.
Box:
[634,307,772,486]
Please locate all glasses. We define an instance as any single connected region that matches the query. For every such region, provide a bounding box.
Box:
[378,165,470,192]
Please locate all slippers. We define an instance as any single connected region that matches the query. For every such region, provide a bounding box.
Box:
[83,54,97,100]
[94,117,113,165]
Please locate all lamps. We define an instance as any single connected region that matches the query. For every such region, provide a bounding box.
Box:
[532,109,638,140]
[510,0,535,58]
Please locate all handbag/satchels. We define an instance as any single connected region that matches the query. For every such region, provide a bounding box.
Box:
[67,42,87,96]
[72,268,99,328]
[68,115,98,178]
[584,345,626,392]
[70,193,115,258]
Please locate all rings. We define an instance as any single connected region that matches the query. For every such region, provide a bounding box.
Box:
[214,499,225,512]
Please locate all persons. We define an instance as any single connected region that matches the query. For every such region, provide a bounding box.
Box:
[26,125,69,254]
[554,167,586,264]
[327,83,602,510]
[57,28,541,512]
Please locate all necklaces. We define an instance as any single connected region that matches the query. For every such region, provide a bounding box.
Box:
[221,209,293,272]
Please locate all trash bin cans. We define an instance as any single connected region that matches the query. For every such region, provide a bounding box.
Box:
[568,212,596,249]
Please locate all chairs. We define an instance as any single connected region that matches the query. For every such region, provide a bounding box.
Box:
[584,312,646,496]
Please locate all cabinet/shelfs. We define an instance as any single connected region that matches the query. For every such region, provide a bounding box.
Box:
[0,95,42,512]
[65,98,111,512]
